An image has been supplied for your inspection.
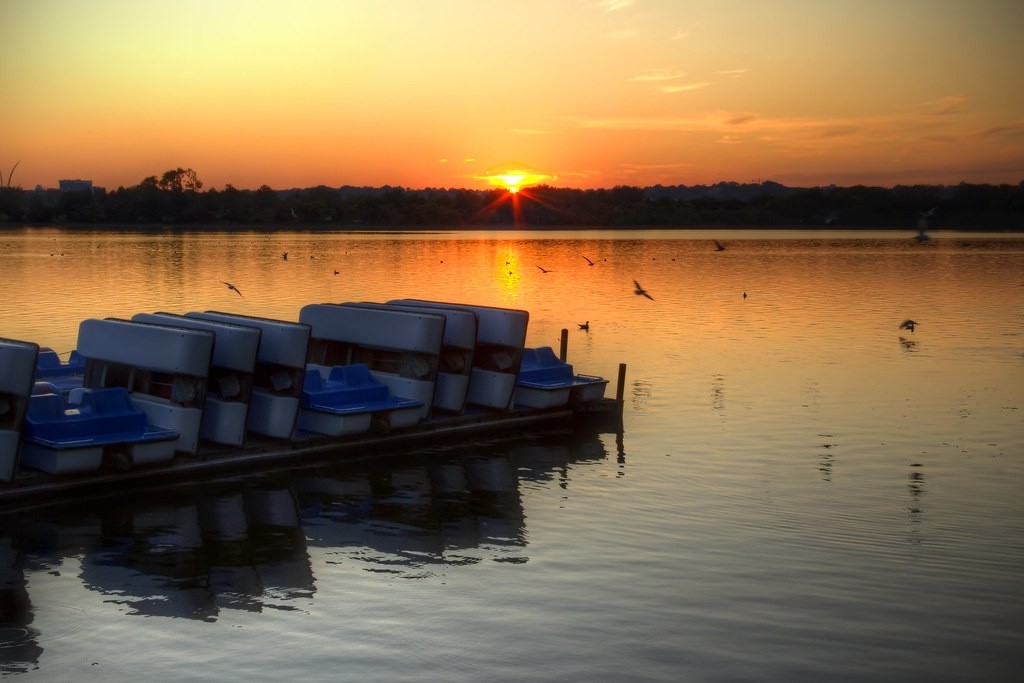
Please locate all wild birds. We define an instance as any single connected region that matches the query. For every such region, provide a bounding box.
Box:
[220,281,243,298]
[652,256,676,262]
[712,239,731,252]
[910,237,930,244]
[505,261,553,276]
[280,244,359,260]
[581,255,609,267]
[333,269,340,276]
[633,278,655,302]
[899,319,918,333]
[578,321,590,329]
[742,291,747,299]
